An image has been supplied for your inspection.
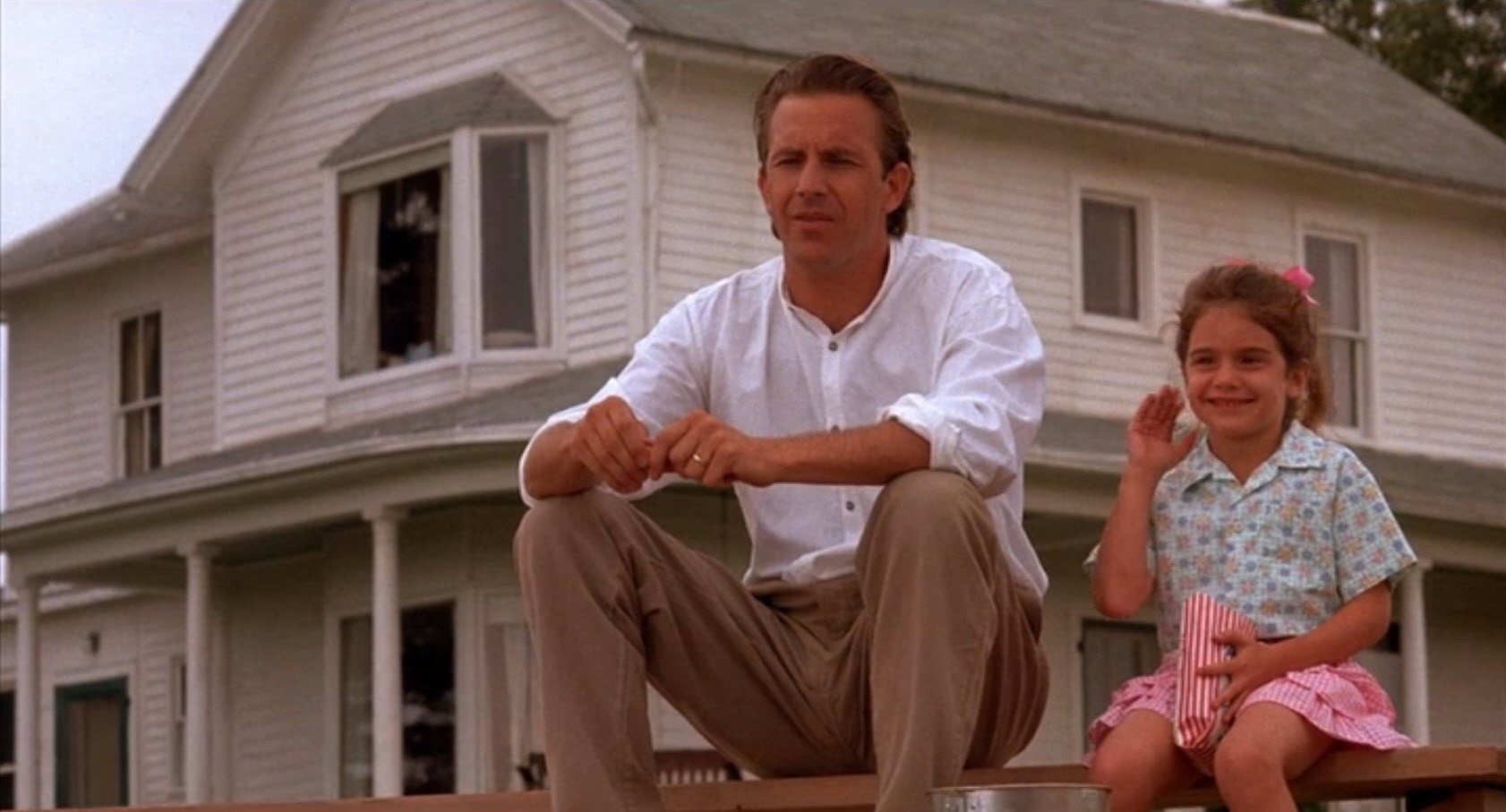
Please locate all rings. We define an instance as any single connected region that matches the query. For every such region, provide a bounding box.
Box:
[691,452,709,465]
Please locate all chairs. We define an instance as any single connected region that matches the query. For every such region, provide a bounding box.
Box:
[654,749,737,782]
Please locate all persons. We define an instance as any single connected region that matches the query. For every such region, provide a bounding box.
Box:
[513,54,1054,812]
[1078,259,1418,812]
